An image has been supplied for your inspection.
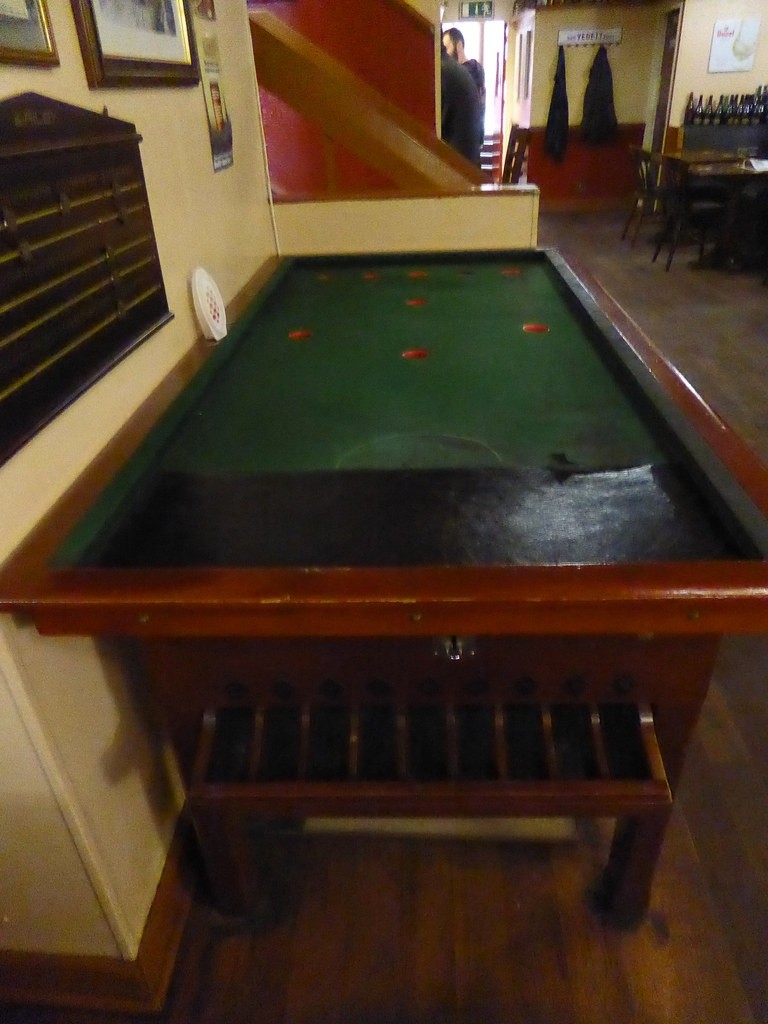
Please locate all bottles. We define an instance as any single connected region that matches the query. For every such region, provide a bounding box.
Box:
[684,85,768,126]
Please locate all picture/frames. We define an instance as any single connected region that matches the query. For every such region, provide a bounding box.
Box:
[0,0,59,67]
[70,0,202,91]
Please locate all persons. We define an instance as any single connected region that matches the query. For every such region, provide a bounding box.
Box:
[441,22,487,170]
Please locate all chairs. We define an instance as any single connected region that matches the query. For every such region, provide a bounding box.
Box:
[502,124,535,185]
[651,154,727,271]
[621,144,677,248]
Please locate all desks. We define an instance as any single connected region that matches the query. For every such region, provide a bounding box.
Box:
[689,159,768,270]
[666,150,746,229]
[0,244,768,931]
